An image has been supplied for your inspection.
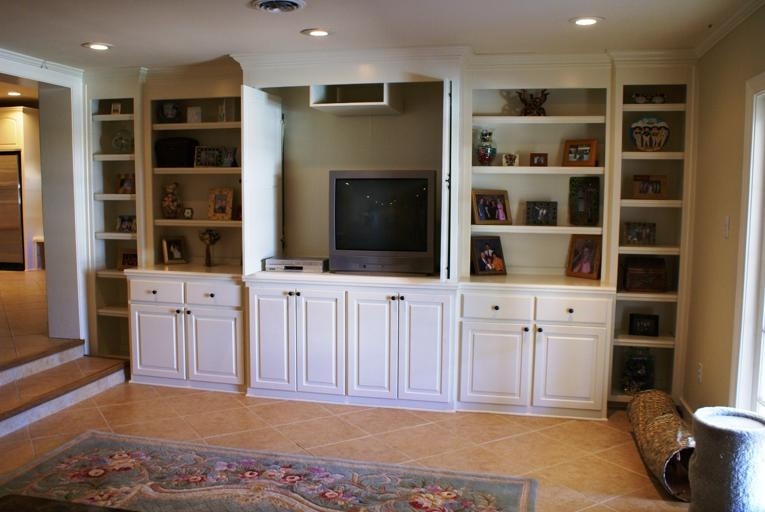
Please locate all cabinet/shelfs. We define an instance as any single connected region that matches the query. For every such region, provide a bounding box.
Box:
[451,269,615,420]
[243,275,346,405]
[144,76,282,276]
[611,68,698,407]
[461,71,613,289]
[348,277,449,412]
[85,84,143,359]
[123,265,249,396]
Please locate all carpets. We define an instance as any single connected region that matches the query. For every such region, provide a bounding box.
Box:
[0,428,538,512]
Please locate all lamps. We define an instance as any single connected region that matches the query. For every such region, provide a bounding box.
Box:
[299,29,335,39]
[567,14,605,31]
[81,42,115,53]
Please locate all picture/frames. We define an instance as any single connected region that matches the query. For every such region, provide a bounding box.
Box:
[471,236,506,277]
[472,188,512,226]
[563,138,597,168]
[161,237,188,265]
[115,213,137,234]
[628,312,661,339]
[208,187,233,222]
[117,248,137,273]
[632,175,669,200]
[622,222,658,246]
[524,200,559,226]
[529,153,549,168]
[565,235,603,280]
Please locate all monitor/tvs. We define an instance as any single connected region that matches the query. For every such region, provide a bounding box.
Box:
[327,169,437,274]
